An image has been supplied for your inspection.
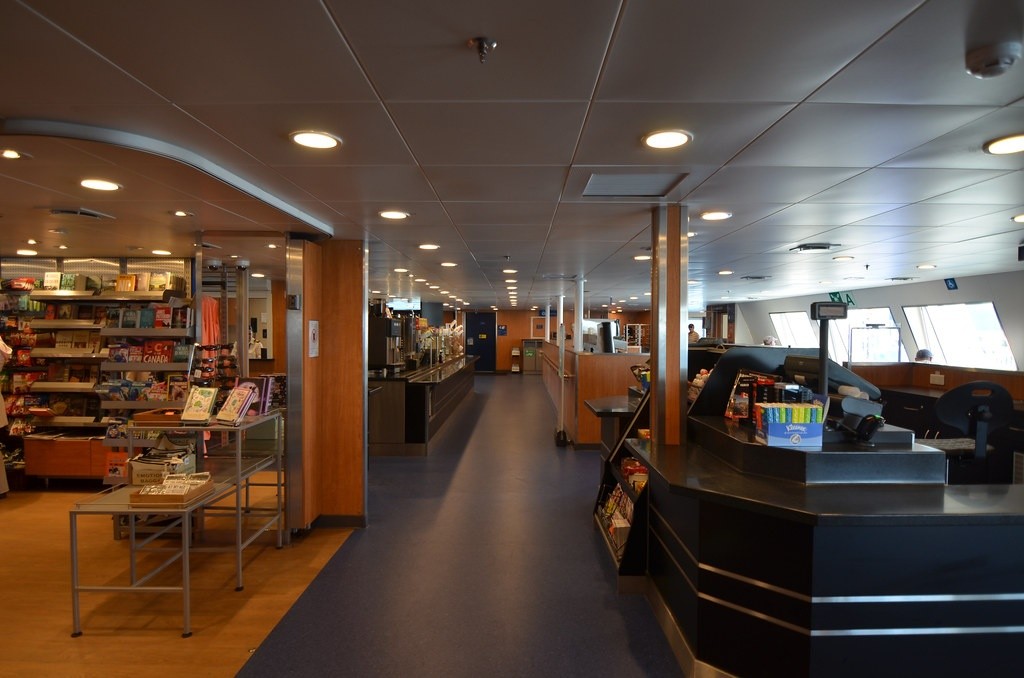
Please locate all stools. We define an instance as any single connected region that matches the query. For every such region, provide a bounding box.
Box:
[914,381,1014,485]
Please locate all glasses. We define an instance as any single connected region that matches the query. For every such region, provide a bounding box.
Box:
[191,343,238,407]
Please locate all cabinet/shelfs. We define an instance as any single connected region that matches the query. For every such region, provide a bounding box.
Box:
[21,290,206,488]
[68,407,284,638]
[368,355,481,457]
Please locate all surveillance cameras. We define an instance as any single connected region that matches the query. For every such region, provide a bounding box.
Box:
[233,260,250,271]
[206,260,222,271]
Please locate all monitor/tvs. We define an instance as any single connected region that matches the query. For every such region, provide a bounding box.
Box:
[783,355,881,400]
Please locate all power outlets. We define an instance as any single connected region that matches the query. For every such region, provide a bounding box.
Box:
[286,294,301,310]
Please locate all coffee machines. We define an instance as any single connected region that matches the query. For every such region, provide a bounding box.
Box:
[369,297,406,374]
[394,308,420,370]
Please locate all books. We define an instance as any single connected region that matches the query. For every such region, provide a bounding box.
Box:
[43,272,197,401]
[216,387,256,425]
[180,386,218,421]
[234,371,288,417]
[755,401,822,443]
[28,408,57,417]
[48,393,105,420]
